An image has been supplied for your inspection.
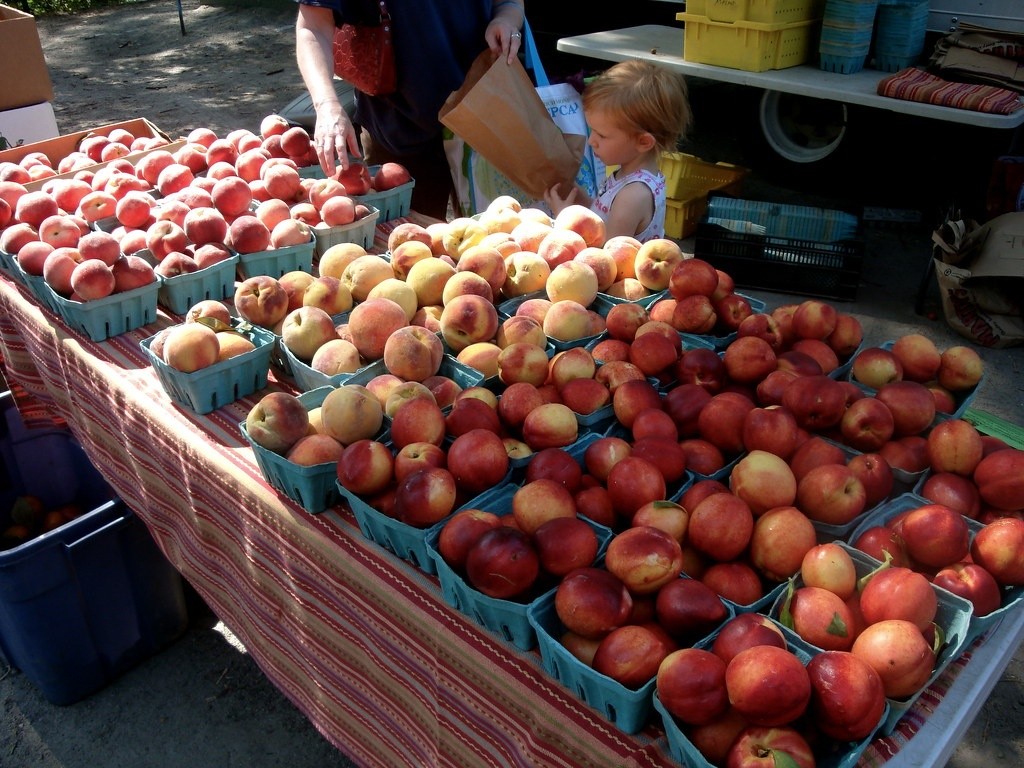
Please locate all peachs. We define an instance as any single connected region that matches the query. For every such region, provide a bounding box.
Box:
[5,498,79,541]
[151,195,685,468]
[338,258,1024,768]
[0,114,411,302]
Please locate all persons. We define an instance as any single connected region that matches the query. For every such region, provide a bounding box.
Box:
[543,59,690,242]
[296,0,525,208]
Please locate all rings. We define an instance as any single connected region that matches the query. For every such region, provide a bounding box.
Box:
[511,33,520,38]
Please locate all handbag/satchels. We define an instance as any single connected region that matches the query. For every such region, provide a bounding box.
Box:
[437,0,606,221]
[327,0,397,96]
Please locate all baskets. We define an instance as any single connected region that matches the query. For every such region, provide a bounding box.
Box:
[0,150,1024,768]
[675,0,929,73]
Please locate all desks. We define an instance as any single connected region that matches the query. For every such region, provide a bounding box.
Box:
[557,25,1024,128]
[0,216,1023,768]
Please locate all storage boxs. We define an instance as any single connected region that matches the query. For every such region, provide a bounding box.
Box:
[0,3,61,151]
[676,0,823,72]
[0,117,1022,768]
[819,0,929,74]
